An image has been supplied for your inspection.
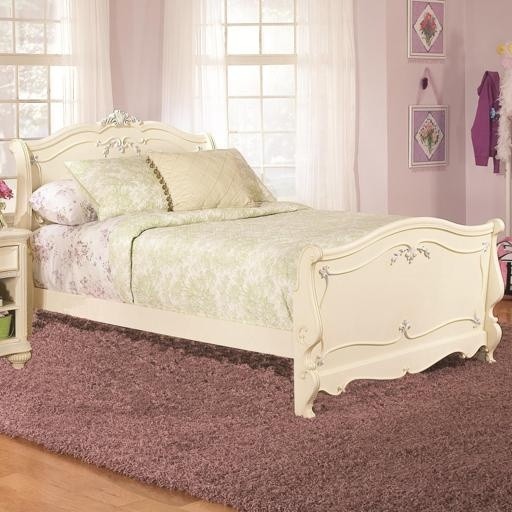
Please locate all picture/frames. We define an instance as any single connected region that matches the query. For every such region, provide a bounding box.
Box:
[407,0,446,59]
[409,104,449,168]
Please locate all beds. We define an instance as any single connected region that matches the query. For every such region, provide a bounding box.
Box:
[9,111,506,421]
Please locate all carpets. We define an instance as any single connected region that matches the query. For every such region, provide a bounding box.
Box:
[0,312,512,511]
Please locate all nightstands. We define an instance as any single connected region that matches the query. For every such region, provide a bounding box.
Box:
[0,226,33,372]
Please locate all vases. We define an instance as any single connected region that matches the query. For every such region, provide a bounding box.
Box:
[0,202,8,232]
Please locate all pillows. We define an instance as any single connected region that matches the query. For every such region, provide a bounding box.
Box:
[65,147,277,219]
[28,180,98,226]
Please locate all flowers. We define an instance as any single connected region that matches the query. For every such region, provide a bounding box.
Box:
[0,180,15,224]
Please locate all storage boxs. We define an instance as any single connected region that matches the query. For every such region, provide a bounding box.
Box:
[0,314,12,338]
